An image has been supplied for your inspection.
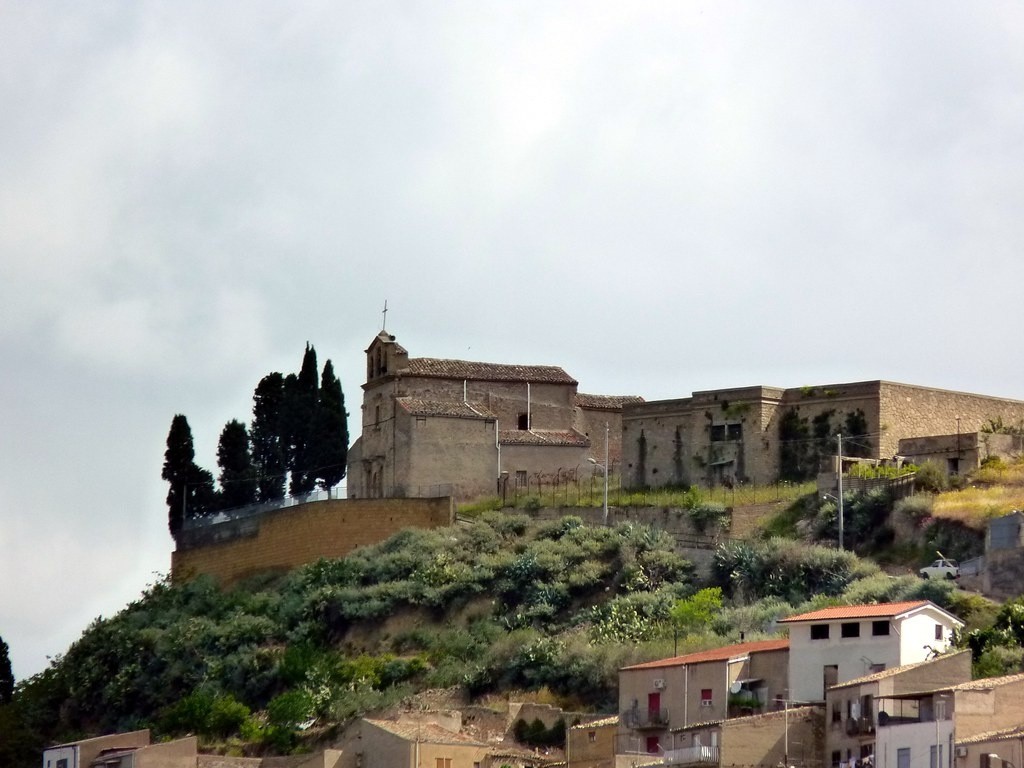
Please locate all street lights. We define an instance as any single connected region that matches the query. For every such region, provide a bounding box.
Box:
[822,492,844,549]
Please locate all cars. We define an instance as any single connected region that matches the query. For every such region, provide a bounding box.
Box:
[919,558,960,580]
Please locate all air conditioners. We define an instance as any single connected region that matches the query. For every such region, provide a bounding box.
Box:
[955,745,966,757]
[653,678,665,689]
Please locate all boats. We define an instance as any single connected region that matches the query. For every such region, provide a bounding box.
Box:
[586,457,609,527]
